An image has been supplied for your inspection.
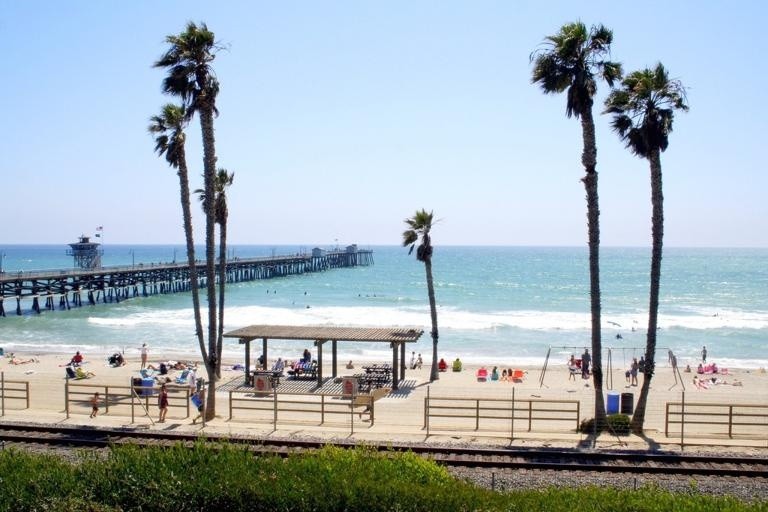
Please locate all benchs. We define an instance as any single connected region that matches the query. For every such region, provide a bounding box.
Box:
[247,360,393,387]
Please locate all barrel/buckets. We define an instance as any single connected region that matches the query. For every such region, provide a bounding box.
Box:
[134,377,142,395]
[621,392,634,414]
[142,379,154,394]
[607,393,620,414]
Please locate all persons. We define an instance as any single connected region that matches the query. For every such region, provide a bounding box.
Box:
[193,388,207,427]
[567,355,578,381]
[439,359,446,371]
[303,349,311,362]
[140,343,200,396]
[346,361,354,369]
[409,352,423,370]
[272,358,284,371]
[630,352,645,386]
[358,386,374,419]
[684,346,742,390]
[68,351,96,379]
[108,352,127,368]
[453,358,462,371]
[478,366,512,381]
[256,355,263,370]
[581,349,591,379]
[158,388,169,423]
[90,392,98,418]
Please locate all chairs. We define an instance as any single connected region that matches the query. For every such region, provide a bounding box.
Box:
[65,355,123,379]
[437,361,522,383]
